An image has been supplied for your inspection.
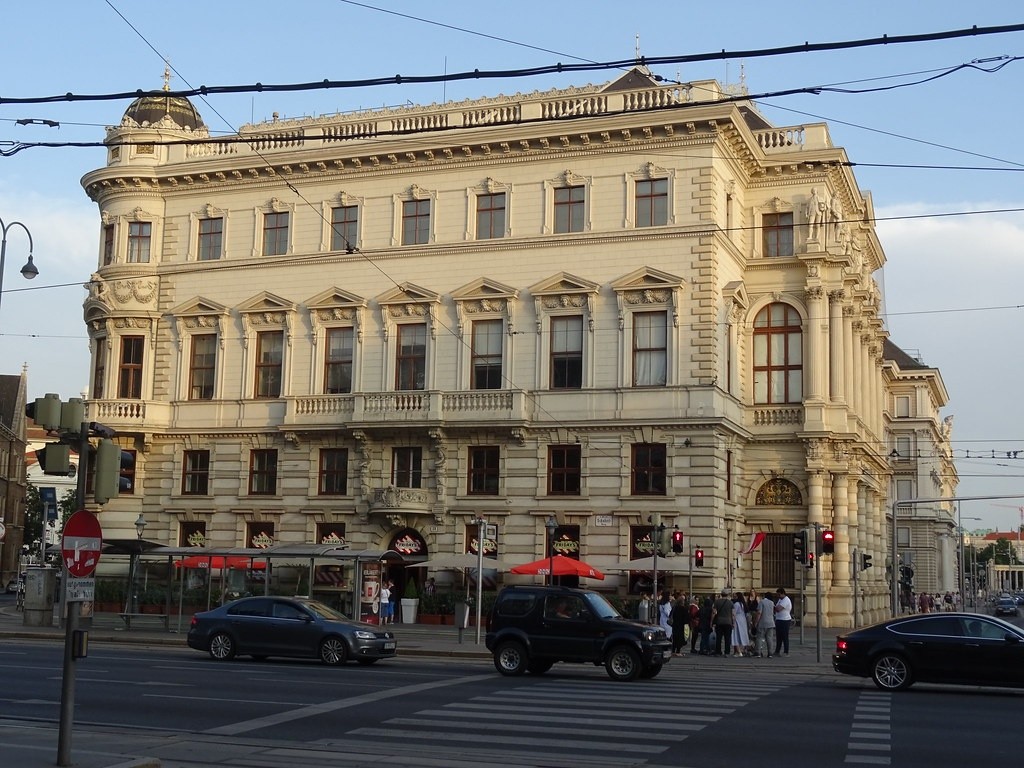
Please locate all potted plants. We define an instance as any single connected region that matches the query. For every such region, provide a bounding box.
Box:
[94,577,222,614]
[418,592,497,626]
[401,577,419,624]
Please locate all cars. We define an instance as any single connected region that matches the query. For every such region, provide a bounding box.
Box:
[985,591,1024,617]
[831,611,1024,693]
[186,594,399,666]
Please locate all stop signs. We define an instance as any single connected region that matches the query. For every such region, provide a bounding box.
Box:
[61,509,104,579]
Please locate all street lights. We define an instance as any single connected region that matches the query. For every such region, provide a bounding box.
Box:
[959,516,983,604]
[0,217,40,308]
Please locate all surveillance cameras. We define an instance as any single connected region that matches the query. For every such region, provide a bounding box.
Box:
[89,422,115,436]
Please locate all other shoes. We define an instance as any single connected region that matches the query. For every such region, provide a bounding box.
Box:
[674,650,790,658]
[387,621,394,624]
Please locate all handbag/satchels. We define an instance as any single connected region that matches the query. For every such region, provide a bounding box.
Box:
[667,619,674,627]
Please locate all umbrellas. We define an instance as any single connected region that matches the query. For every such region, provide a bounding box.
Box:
[510,553,604,588]
[607,555,715,575]
[404,551,512,598]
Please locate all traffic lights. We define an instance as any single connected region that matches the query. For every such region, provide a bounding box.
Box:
[806,552,814,568]
[673,532,683,553]
[862,553,873,569]
[793,531,807,564]
[822,530,835,554]
[695,550,703,566]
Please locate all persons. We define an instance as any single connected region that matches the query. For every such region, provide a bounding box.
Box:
[901,590,960,614]
[380,579,396,626]
[548,598,571,619]
[651,585,793,658]
[425,578,438,597]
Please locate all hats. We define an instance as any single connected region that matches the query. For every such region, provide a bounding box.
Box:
[721,589,728,595]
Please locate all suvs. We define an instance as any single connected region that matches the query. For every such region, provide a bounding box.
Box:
[485,583,674,683]
